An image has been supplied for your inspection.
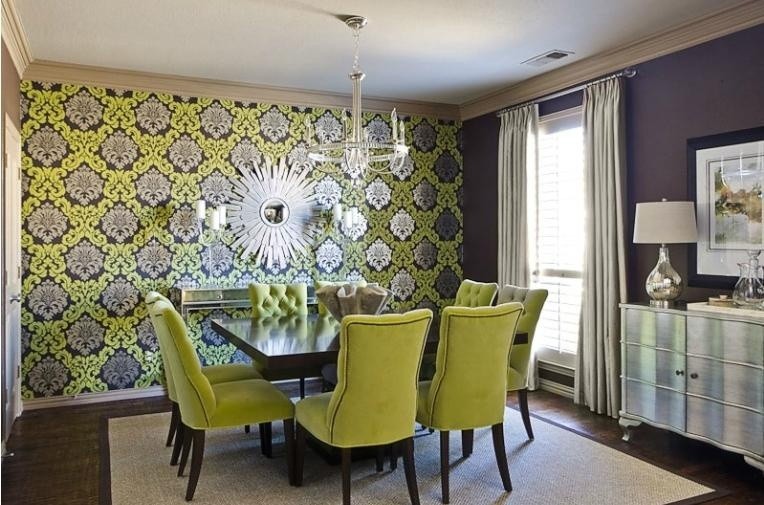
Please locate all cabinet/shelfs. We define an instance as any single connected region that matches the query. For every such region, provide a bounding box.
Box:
[617,300,686,446]
[687,295,764,477]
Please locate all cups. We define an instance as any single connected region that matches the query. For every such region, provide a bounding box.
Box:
[720,294,728,300]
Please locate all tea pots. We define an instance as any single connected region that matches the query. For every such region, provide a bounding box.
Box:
[732,263,764,307]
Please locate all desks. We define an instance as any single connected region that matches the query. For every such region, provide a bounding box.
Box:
[207,311,526,487]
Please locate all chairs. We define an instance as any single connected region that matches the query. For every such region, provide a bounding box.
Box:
[449,277,497,310]
[152,297,297,501]
[415,297,524,502]
[143,289,265,469]
[491,285,548,438]
[295,309,433,504]
[248,283,310,321]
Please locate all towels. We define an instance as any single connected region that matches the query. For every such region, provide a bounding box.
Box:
[315,284,395,320]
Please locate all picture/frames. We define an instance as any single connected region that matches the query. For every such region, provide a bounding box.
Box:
[683,126,763,290]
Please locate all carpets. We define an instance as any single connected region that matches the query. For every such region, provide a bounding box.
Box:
[96,395,715,504]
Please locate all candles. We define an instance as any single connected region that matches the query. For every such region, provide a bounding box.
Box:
[333,203,361,230]
[216,205,228,225]
[210,212,219,231]
[193,198,207,221]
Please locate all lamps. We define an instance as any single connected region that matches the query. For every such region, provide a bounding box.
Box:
[304,14,414,186]
[632,197,699,313]
